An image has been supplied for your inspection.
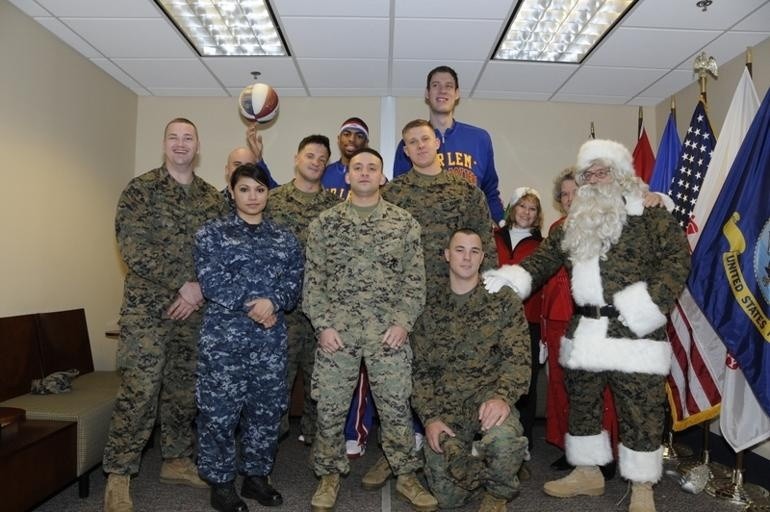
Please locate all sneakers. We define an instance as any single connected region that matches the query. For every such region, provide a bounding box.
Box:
[544,465,605,498]
[105,473,133,512]
[159,457,282,512]
[629,483,655,512]
[311,433,438,512]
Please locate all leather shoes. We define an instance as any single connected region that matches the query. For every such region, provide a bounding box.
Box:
[551,455,616,480]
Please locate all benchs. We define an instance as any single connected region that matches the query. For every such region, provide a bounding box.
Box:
[0,307,153,512]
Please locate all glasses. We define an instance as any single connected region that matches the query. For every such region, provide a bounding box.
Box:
[582,170,606,180]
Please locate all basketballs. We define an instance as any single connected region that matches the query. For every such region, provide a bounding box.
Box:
[239,83,279,124]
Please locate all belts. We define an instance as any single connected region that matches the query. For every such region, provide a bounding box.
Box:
[574,303,618,319]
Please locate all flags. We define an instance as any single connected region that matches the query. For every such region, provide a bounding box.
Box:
[630,66,770,454]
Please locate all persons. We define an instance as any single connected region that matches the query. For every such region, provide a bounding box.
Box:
[360,117,499,492]
[392,65,505,451]
[245,116,388,458]
[220,147,258,208]
[408,226,534,511]
[493,187,546,482]
[301,146,440,510]
[100,117,229,512]
[263,134,345,446]
[191,164,305,511]
[483,139,692,512]
[540,166,675,483]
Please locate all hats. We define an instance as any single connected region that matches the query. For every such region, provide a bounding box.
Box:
[509,187,539,206]
